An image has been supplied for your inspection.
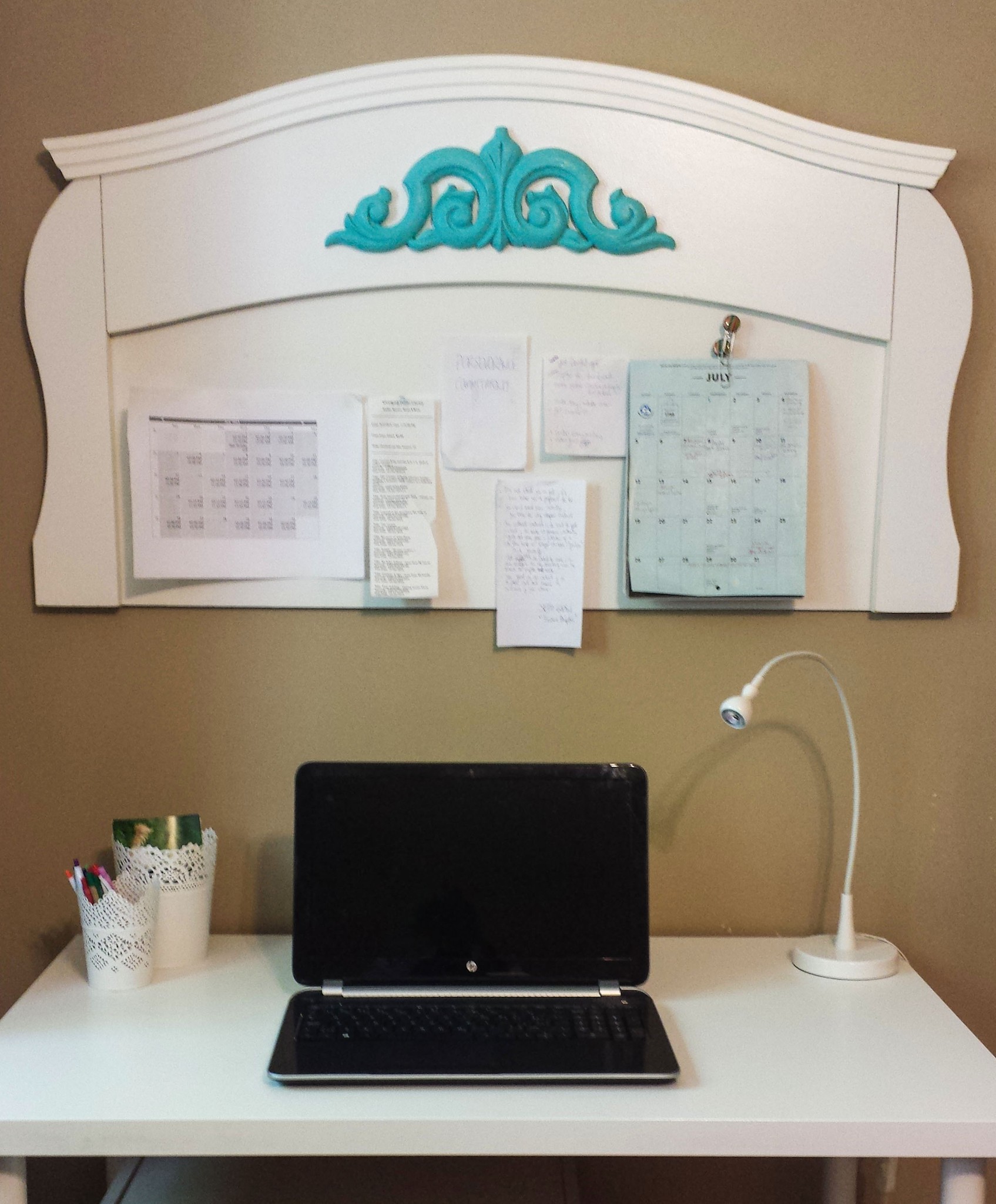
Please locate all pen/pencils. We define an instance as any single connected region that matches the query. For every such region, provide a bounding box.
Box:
[65,858,116,906]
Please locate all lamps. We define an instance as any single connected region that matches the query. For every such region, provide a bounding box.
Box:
[717,650,902,979]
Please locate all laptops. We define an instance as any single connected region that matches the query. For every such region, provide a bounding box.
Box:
[261,761,683,1091]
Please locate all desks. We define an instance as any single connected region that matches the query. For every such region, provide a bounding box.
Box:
[0,932,996,1203]
